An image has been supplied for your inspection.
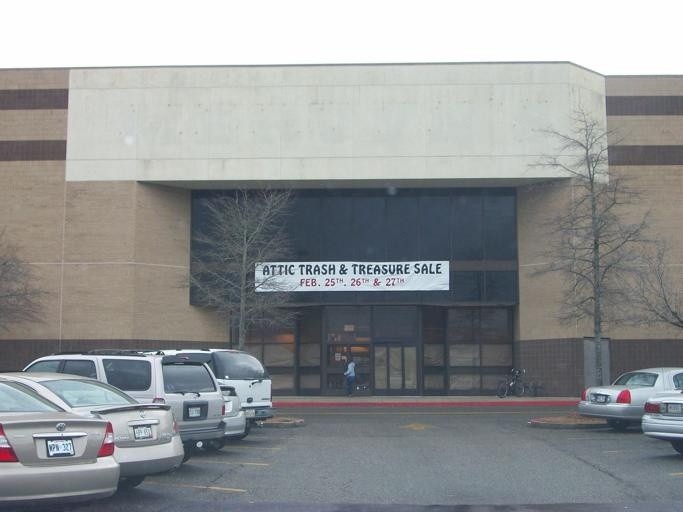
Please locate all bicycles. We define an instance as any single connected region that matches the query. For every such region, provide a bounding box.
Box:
[497,367,527,398]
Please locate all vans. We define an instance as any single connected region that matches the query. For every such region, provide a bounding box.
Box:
[137,345,276,421]
[22,349,227,462]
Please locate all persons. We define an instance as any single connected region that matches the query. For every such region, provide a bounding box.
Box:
[344,358,355,397]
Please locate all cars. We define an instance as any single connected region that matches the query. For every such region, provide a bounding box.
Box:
[0,370,185,512]
[577,366,682,429]
[198,381,248,451]
[641,389,683,454]
